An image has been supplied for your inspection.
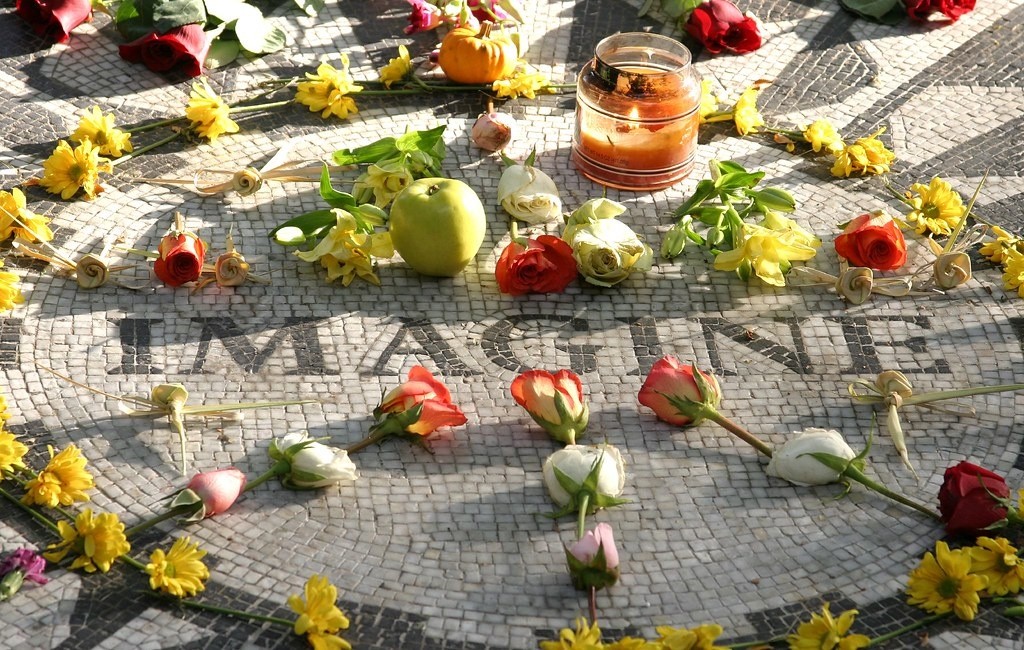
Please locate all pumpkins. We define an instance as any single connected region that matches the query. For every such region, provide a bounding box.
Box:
[439,20,517,83]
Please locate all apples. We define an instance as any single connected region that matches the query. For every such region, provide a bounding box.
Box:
[389,177,486,278]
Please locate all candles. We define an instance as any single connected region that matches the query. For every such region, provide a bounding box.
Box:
[572,32,702,191]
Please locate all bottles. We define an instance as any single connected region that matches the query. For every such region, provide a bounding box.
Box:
[571,32,701,190]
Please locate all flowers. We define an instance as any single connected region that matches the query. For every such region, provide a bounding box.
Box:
[0,0,1024,650]
